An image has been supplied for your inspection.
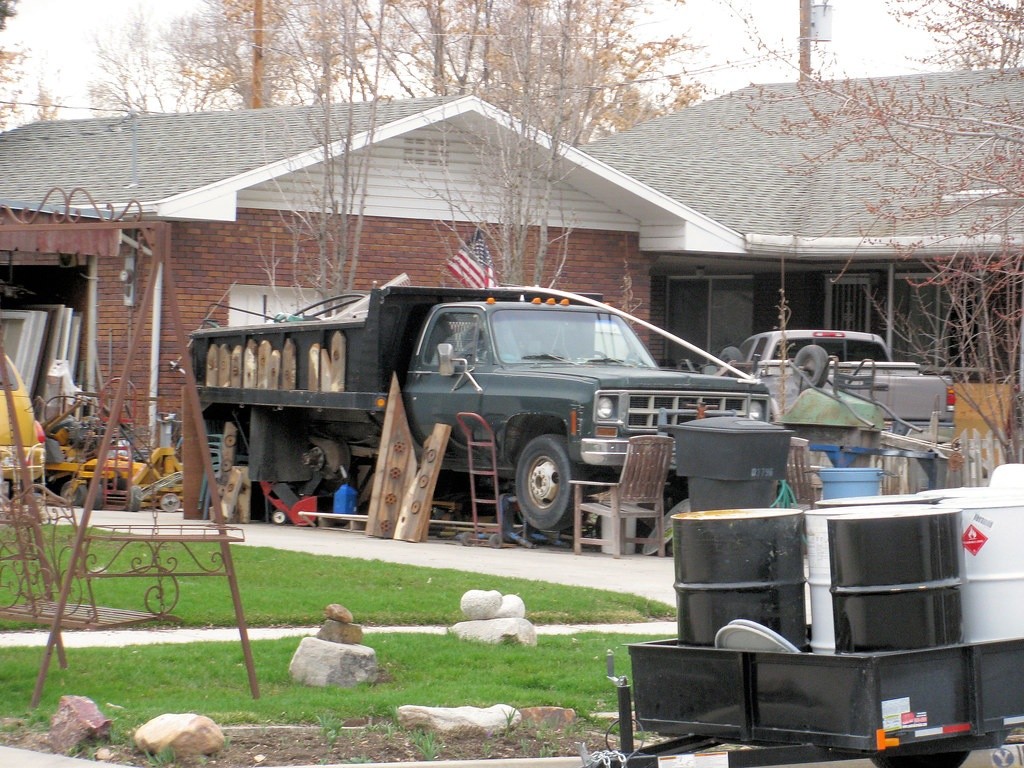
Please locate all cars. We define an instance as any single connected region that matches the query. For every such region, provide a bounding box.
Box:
[0,351,47,499]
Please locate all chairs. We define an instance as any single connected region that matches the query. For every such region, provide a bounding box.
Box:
[787,437,814,510]
[568,435,676,560]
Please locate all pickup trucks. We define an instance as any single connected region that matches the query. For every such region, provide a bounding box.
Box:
[738,329,957,446]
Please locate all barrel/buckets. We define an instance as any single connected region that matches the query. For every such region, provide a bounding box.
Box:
[803,487,1024,653]
[669,508,810,648]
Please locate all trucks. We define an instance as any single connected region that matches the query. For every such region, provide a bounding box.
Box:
[186,281,770,532]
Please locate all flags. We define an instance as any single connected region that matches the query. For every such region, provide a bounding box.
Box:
[447,226,499,287]
